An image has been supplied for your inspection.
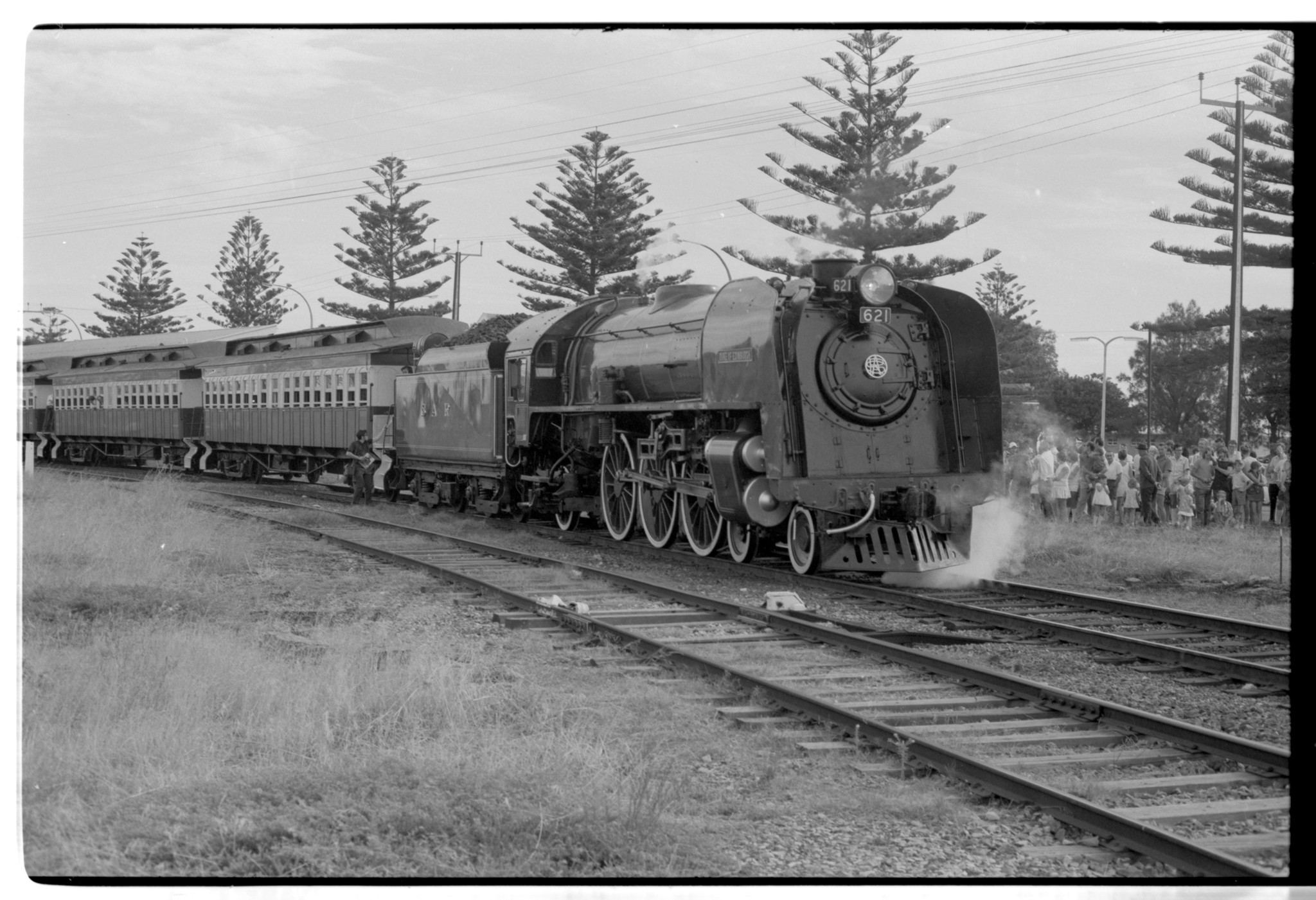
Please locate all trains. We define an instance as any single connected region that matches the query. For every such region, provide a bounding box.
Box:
[24,255,1009,577]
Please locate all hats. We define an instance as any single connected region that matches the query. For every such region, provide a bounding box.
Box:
[1008,442,1018,449]
[1135,443,1149,450]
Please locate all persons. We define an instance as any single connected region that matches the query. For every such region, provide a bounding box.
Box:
[345,424,388,507]
[1003,425,1292,530]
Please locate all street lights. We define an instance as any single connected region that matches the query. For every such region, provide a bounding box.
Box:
[260,282,314,329]
[1068,335,1146,453]
[671,236,734,283]
